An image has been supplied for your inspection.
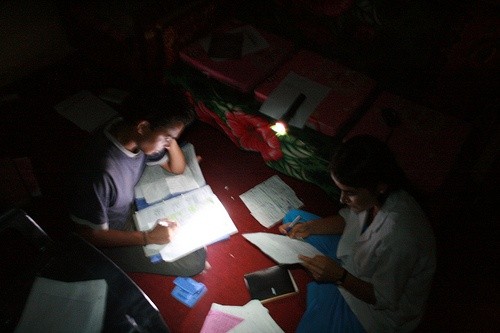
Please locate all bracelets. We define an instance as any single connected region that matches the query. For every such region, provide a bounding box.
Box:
[142,231,148,247]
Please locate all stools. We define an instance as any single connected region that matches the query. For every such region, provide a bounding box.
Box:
[180,15,472,193]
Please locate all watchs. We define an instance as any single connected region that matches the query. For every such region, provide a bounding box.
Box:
[337,269,347,285]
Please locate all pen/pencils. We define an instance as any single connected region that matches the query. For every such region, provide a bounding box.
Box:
[281,215,301,236]
[159,221,169,227]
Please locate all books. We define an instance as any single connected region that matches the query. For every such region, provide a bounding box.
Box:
[133,143,238,264]
[244,264,299,304]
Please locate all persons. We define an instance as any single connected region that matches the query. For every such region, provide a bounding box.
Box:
[277,133,437,333]
[60,84,212,276]
